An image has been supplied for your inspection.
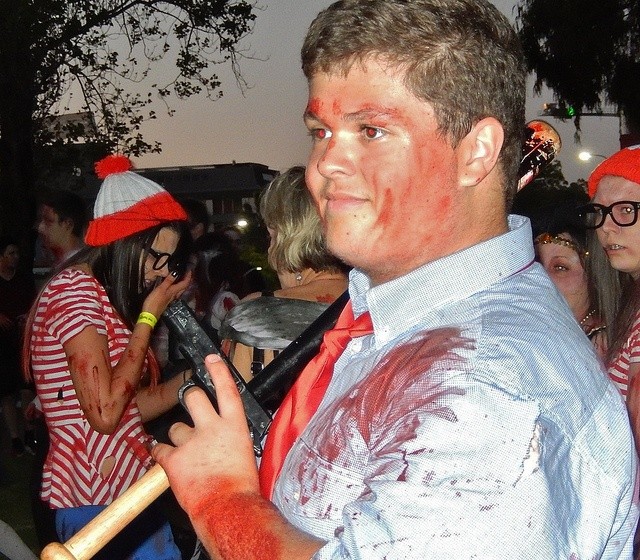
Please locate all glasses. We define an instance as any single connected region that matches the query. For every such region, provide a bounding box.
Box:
[575,200,640,230]
[146,245,173,271]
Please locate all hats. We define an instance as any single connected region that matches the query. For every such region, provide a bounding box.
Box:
[84,155,189,247]
[587,145,640,197]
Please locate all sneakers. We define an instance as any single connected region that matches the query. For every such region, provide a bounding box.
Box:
[12,444,25,458]
[25,440,37,456]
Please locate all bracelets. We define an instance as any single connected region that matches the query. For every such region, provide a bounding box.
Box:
[136,310,158,330]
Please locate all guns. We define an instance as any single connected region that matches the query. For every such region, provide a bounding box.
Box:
[163,300,273,456]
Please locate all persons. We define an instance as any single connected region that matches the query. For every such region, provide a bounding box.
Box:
[21,190,86,549]
[532,216,611,366]
[191,166,354,559]
[150,0,639,560]
[193,232,265,348]
[578,143,640,464]
[179,198,218,317]
[20,154,194,560]
[221,209,282,297]
[1,240,41,459]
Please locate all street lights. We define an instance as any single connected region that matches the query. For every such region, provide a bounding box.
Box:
[578,151,607,162]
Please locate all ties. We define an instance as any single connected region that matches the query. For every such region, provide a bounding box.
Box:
[258,300,374,502]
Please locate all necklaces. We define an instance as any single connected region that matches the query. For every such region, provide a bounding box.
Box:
[579,308,598,326]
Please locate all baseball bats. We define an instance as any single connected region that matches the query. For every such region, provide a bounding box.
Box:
[41,120,561,559]
